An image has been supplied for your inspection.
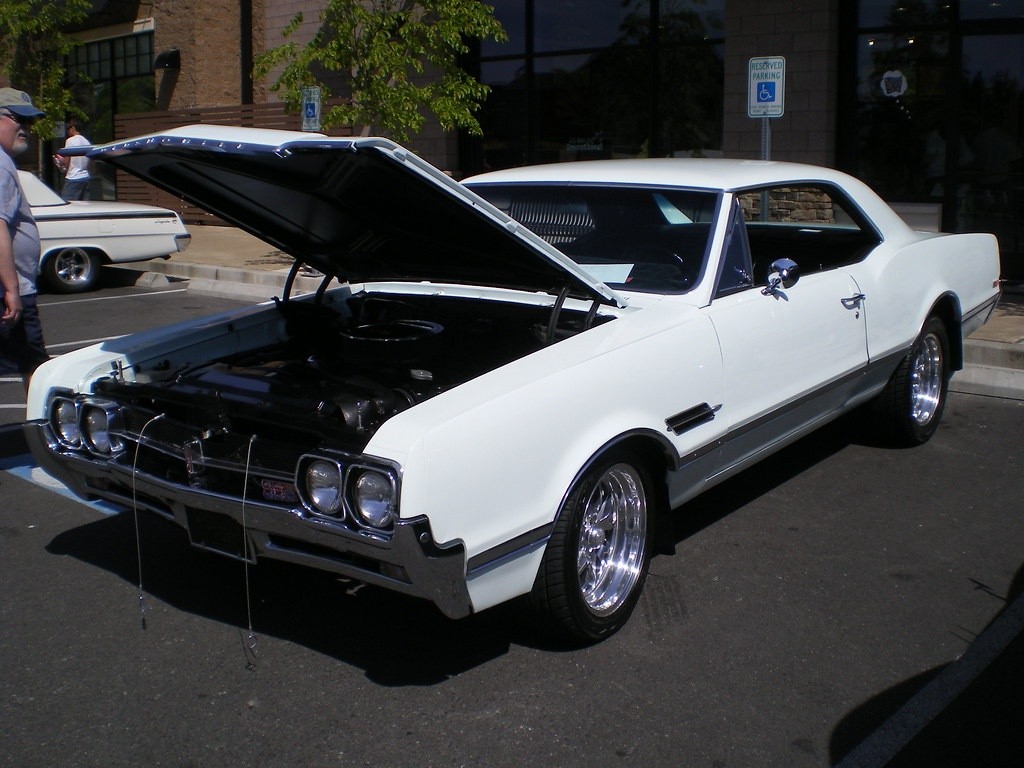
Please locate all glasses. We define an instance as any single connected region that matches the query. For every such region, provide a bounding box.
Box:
[0,113,35,126]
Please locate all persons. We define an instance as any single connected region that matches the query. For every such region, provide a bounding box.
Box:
[0,86,52,400]
[57,119,95,201]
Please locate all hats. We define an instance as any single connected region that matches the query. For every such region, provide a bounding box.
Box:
[0,87,46,119]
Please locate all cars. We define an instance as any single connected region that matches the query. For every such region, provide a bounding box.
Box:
[13,169,191,295]
[20,122,1005,648]
